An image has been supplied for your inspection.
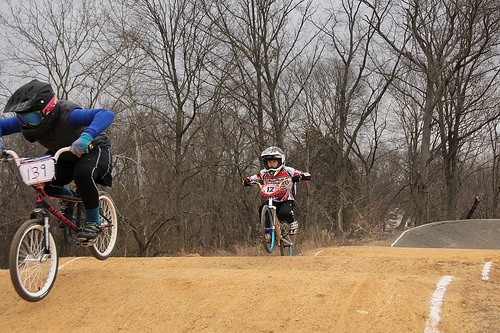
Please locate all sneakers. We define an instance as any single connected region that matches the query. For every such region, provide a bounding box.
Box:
[58,190,78,229]
[77,221,102,241]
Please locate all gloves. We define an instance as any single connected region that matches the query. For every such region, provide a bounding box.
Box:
[243,179,251,186]
[0,137,5,158]
[292,174,303,183]
[69,132,93,156]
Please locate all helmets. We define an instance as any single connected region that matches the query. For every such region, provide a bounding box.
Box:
[4,78,59,143]
[261,146,285,177]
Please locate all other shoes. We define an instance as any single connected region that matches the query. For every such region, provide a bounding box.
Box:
[260,234,271,243]
[289,221,298,236]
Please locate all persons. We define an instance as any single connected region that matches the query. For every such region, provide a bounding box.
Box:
[0,79,115,243]
[241,146,310,244]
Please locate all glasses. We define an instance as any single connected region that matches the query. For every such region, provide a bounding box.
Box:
[16,97,58,127]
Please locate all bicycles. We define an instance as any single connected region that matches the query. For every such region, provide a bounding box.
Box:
[248,172,302,258]
[0,141,119,305]
